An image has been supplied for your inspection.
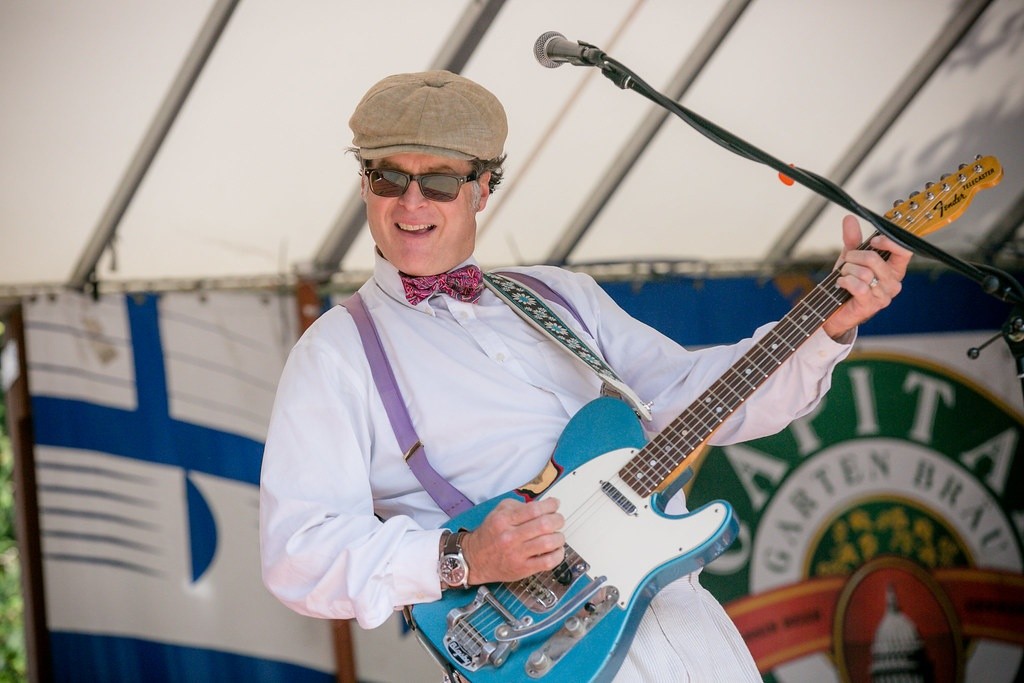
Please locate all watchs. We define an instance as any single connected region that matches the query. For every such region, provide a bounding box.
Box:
[438,527,472,591]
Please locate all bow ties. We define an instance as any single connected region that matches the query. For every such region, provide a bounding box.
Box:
[398,265,484,305]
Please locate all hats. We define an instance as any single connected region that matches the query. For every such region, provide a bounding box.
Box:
[348,70,508,161]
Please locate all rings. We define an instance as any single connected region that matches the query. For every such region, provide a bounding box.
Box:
[869,277,879,287]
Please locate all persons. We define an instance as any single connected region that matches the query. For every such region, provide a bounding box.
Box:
[260,70,914,683]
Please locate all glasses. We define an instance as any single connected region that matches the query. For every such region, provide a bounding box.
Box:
[363,167,479,203]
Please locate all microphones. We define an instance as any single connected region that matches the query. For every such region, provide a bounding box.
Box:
[532,30,606,68]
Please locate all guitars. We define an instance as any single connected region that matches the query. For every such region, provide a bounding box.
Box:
[402,153,1005,683]
